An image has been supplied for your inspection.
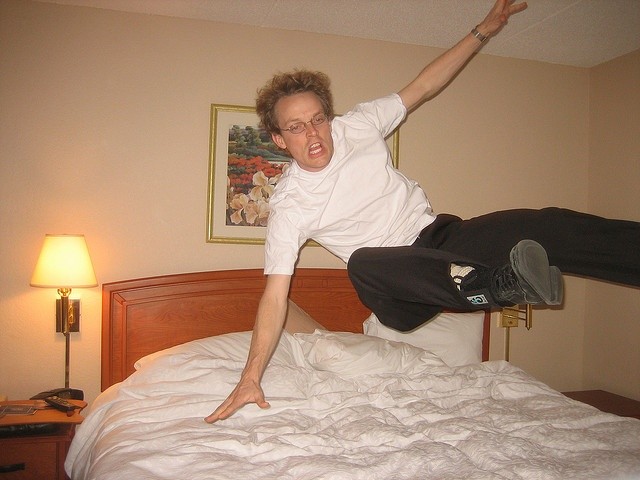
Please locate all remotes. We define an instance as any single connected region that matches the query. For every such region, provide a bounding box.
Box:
[45,396,75,411]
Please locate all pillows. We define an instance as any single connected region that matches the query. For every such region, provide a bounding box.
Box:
[363,311,486,367]
[135,329,316,369]
[283,298,328,334]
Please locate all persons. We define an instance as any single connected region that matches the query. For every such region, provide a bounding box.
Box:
[205,0,640,423]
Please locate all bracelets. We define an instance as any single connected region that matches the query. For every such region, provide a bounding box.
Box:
[471,29,487,43]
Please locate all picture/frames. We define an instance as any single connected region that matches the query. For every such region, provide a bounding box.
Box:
[205,103,399,246]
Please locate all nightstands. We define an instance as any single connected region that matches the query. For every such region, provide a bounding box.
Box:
[0,399,88,479]
[563,385,640,418]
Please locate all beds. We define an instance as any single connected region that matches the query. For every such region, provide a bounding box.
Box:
[65,268,640,480]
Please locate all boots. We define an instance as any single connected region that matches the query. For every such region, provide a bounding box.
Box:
[453,238,564,309]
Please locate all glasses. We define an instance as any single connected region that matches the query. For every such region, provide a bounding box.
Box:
[279,112,325,134]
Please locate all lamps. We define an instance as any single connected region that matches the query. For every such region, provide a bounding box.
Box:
[30,234,98,388]
